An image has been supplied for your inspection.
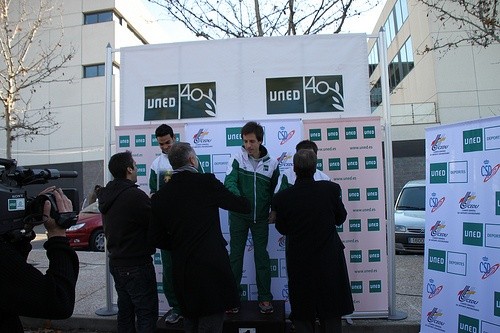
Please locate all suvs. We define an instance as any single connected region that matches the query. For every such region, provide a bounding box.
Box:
[395,180,426,254]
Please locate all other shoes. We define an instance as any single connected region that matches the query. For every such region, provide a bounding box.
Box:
[165,313,183,323]
[258,301,274,313]
[225,306,240,313]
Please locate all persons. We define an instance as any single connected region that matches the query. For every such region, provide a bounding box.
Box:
[89,184,102,205]
[224,121,280,314]
[150,142,252,333]
[0,185,80,333]
[95,149,159,333]
[266,140,355,333]
[148,124,205,323]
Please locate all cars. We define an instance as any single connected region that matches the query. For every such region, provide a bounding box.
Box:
[65,199,106,252]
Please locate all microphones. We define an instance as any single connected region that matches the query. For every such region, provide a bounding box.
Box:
[40,169,78,180]
